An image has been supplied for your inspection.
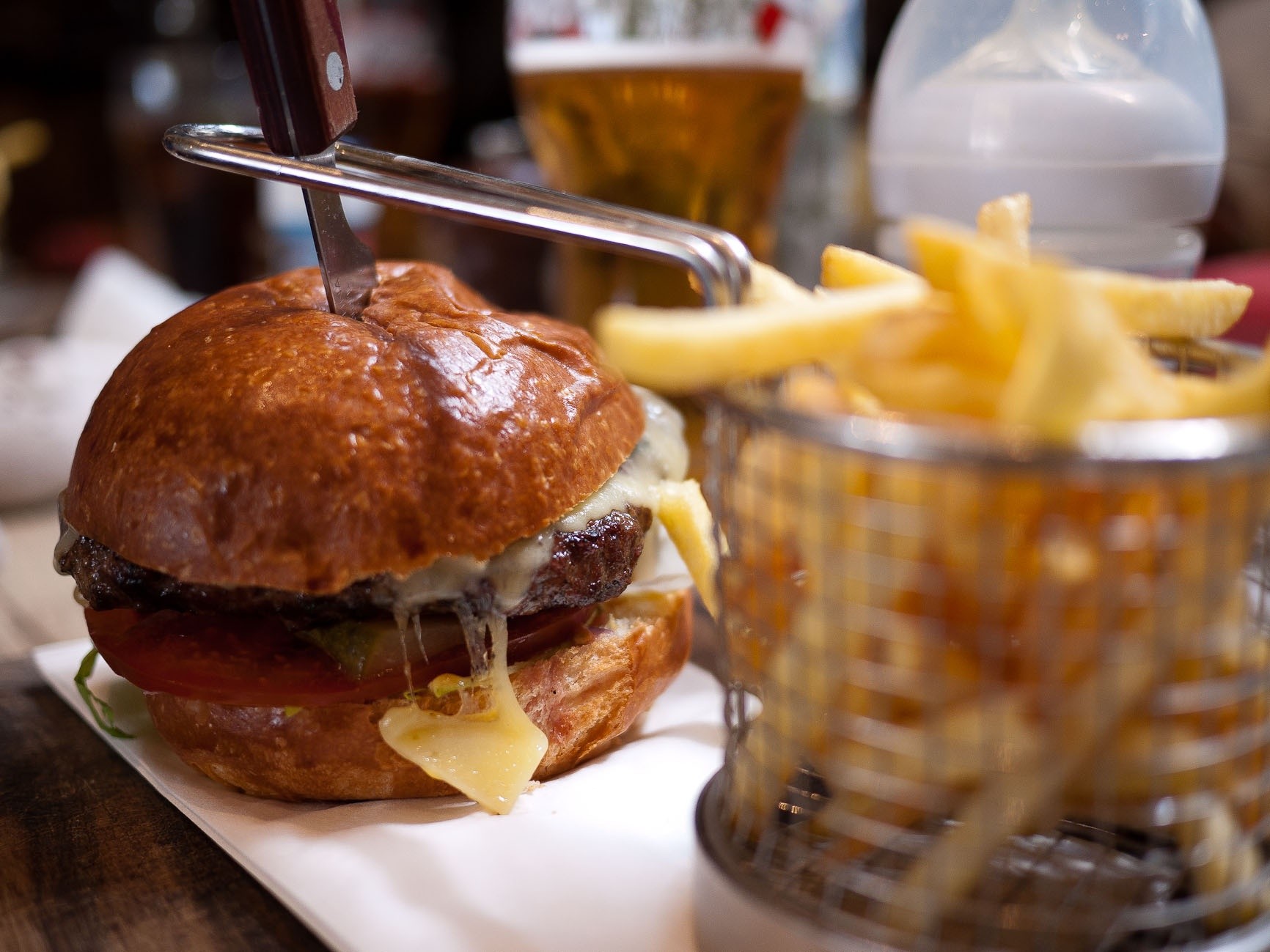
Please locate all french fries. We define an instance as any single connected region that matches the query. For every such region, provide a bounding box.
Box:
[590,190,1270,934]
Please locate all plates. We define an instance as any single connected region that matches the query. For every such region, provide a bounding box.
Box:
[34,631,1269,952]
[1,247,195,507]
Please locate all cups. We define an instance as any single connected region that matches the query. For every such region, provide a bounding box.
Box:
[0,0,200,343]
[509,1,823,483]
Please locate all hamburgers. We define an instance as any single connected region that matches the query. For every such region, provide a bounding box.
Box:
[52,261,718,814]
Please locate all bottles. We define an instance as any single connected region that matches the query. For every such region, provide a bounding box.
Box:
[863,0,1225,280]
